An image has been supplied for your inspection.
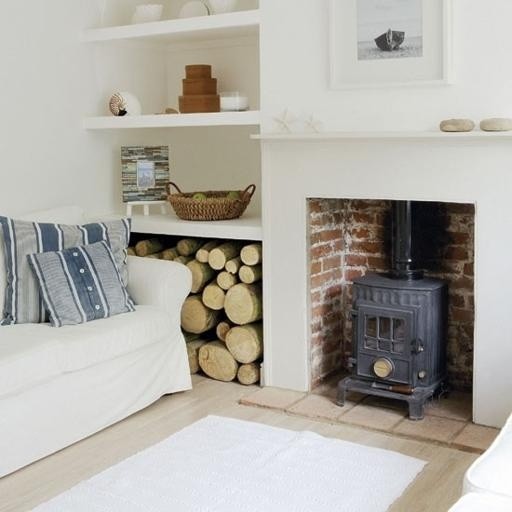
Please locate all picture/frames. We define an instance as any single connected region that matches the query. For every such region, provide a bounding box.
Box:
[332,0,454,88]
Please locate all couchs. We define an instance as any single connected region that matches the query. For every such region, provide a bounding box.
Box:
[0,247,192,479]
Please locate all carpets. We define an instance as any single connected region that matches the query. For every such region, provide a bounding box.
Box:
[28,416,432,512]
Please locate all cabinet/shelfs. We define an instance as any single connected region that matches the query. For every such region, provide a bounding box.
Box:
[82,0,329,394]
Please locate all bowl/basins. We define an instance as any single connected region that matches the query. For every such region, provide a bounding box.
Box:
[218,89,250,112]
[136,3,163,22]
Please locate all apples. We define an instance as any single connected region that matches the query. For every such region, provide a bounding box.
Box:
[228,191,241,198]
[192,193,206,200]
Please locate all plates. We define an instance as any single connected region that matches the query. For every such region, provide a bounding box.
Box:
[177,3,208,19]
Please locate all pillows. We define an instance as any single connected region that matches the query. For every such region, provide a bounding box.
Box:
[4,216,136,328]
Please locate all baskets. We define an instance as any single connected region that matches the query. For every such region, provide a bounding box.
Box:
[165,182,256,221]
